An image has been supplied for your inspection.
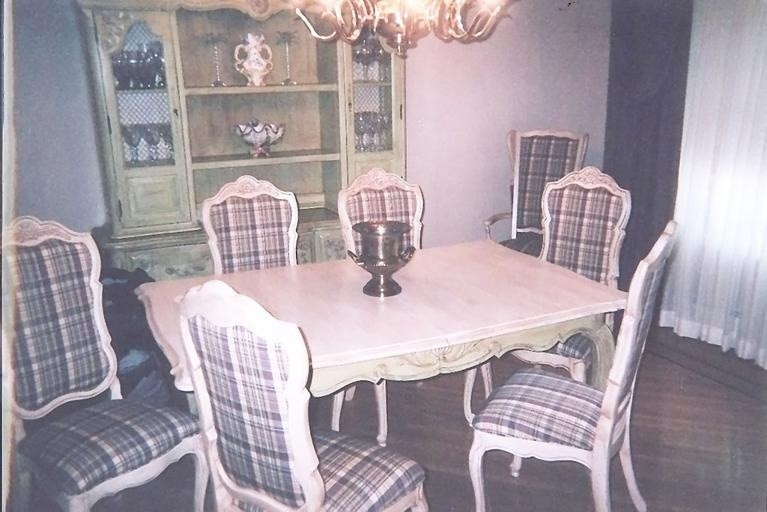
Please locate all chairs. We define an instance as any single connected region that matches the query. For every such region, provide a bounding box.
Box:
[467,221,676,510]
[480,166,632,402]
[176,281,429,511]
[200,174,344,431]
[483,129,588,260]
[1,214,210,510]
[337,169,484,447]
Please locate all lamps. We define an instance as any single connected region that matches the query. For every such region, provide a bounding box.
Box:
[295,0,521,57]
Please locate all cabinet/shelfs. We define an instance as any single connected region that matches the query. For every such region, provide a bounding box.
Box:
[75,0,407,283]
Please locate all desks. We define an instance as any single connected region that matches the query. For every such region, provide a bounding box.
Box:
[135,240,629,415]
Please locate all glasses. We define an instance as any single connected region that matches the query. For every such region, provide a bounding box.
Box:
[295,0,521,57]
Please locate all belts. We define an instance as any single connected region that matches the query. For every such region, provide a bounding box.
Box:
[110,40,174,167]
[352,35,392,154]
